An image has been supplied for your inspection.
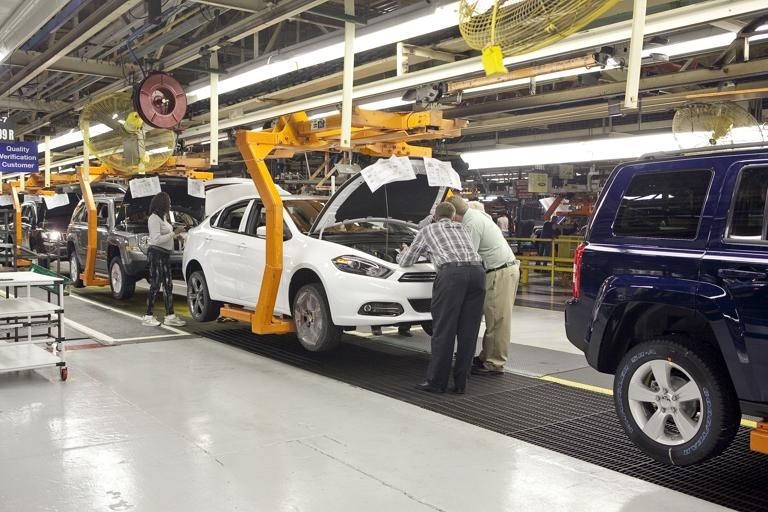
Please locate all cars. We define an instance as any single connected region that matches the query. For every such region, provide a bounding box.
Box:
[181,156,474,353]
[6,192,83,270]
[0,193,15,254]
[469,191,596,249]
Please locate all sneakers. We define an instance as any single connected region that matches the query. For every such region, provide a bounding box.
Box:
[163,314,186,327]
[141,315,162,327]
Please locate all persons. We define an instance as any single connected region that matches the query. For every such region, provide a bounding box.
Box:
[534,216,562,273]
[141,192,186,328]
[496,210,508,237]
[395,202,487,394]
[445,196,521,375]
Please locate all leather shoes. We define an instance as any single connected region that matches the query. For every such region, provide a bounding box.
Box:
[414,378,448,395]
[470,356,506,376]
[449,383,467,395]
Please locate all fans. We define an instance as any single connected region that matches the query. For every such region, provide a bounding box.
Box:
[666,99,768,154]
[452,1,621,78]
[76,86,180,176]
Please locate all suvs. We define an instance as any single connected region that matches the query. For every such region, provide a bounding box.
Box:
[565,141,768,467]
[66,174,205,300]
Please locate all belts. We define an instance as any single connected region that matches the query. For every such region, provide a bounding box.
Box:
[485,260,519,275]
[437,260,484,270]
